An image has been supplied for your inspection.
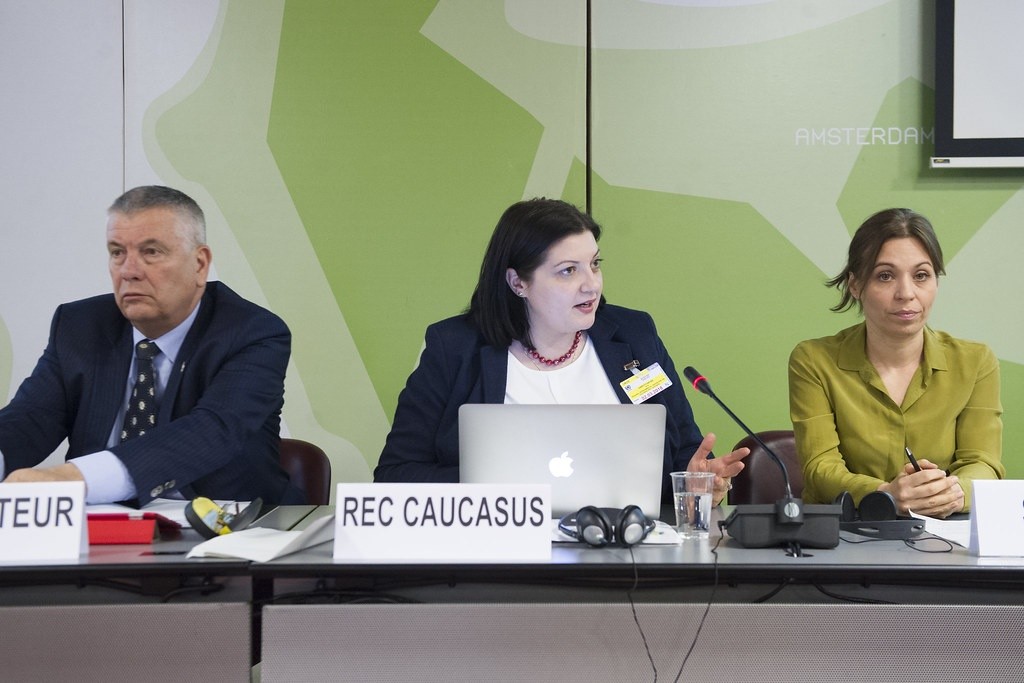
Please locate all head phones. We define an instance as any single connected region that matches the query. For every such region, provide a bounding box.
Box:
[558,506,656,548]
[835,490,925,540]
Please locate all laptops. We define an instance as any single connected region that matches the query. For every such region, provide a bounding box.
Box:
[457,403,666,520]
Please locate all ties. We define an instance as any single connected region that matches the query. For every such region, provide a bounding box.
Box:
[118,338,159,444]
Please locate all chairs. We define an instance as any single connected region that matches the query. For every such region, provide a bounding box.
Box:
[728,428,806,509]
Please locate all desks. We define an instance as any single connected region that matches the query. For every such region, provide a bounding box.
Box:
[0,505,1024,683]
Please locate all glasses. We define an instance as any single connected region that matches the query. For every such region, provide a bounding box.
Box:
[215,500,240,529]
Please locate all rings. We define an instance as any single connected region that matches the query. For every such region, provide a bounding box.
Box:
[723,478,733,490]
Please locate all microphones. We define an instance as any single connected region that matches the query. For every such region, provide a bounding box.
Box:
[683,366,843,547]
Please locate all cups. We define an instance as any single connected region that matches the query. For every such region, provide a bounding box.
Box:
[670,471,716,540]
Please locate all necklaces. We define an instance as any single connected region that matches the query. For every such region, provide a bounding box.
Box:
[522,331,582,366]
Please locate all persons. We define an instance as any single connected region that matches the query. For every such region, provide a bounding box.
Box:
[786,207,1004,520]
[373,196,752,525]
[0,184,293,510]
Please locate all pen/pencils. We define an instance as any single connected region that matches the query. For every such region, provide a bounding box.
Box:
[901,446,921,472]
